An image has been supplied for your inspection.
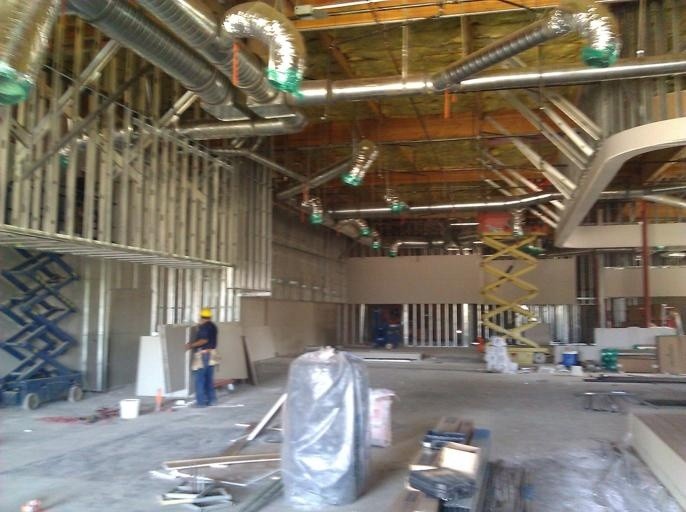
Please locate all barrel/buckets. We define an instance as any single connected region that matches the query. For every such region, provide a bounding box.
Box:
[119,399,141,419]
[561,350,578,365]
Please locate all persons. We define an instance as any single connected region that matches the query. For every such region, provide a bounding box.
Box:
[181,307,220,409]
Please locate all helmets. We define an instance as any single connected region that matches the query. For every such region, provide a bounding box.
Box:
[200,309,213,319]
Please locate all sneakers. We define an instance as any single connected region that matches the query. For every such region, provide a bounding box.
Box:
[188,398,219,409]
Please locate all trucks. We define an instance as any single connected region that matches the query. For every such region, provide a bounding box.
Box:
[371,307,401,350]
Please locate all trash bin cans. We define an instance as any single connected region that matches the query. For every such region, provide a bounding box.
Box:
[562,351,579,368]
